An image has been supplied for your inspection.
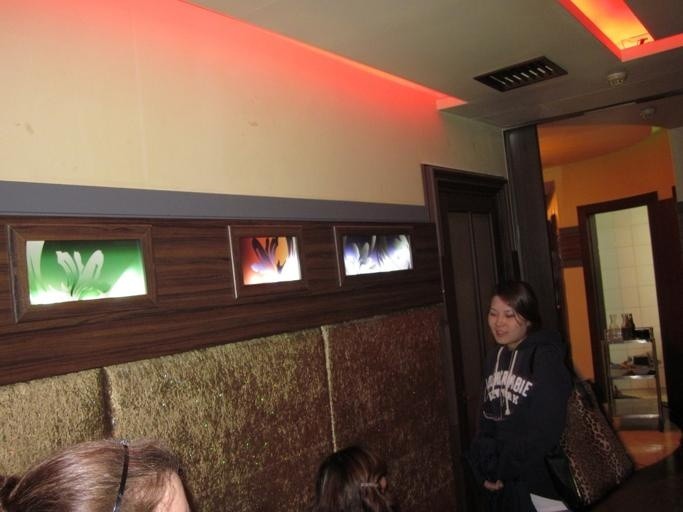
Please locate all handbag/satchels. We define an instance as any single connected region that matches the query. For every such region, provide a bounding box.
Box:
[558,381,637,510]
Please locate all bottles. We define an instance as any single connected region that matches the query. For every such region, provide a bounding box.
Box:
[609,313,635,340]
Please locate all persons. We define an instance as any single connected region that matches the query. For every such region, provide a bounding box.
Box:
[463,278,576,512]
[1,439,191,511]
[315,446,394,512]
